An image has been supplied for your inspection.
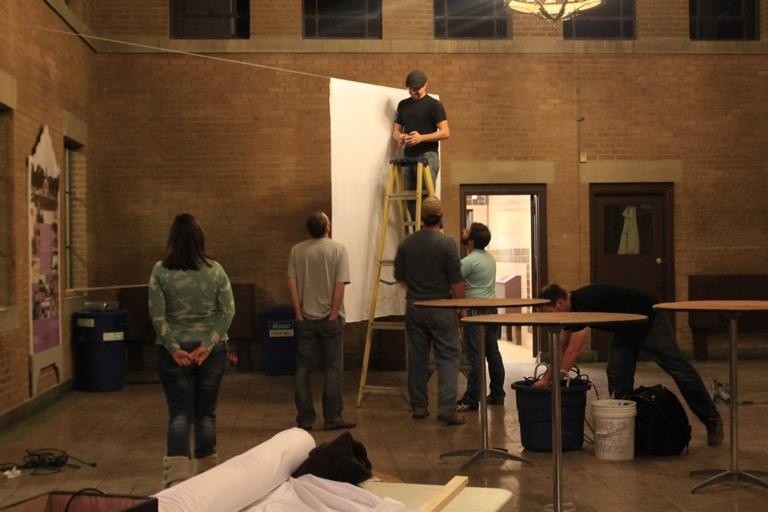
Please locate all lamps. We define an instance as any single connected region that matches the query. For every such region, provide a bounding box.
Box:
[504,0,606,23]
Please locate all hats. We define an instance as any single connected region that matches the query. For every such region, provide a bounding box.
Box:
[405,70,427,87]
[421,197,443,222]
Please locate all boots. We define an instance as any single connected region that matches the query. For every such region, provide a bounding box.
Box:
[163,456,188,487]
[193,453,218,476]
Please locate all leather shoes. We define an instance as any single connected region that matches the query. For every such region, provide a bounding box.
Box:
[437,410,466,424]
[298,422,313,430]
[413,406,430,421]
[706,416,724,446]
[324,417,356,430]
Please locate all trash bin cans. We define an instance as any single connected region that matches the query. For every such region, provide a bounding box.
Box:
[256,306,300,377]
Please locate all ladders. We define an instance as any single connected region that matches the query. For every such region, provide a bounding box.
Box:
[356,157,471,409]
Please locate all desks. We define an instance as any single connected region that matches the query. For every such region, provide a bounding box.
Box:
[414,299,550,472]
[461,313,648,512]
[653,301,768,494]
[360,483,513,512]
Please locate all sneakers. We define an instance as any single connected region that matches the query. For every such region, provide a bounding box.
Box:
[486,394,504,406]
[454,399,478,412]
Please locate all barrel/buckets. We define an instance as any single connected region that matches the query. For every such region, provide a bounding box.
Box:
[508,377,592,454]
[261,306,302,379]
[587,398,640,462]
[230,280,257,362]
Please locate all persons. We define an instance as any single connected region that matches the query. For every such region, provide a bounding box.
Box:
[458,220,506,412]
[149,212,235,490]
[393,196,469,426]
[393,71,451,234]
[287,213,358,431]
[533,283,724,448]
